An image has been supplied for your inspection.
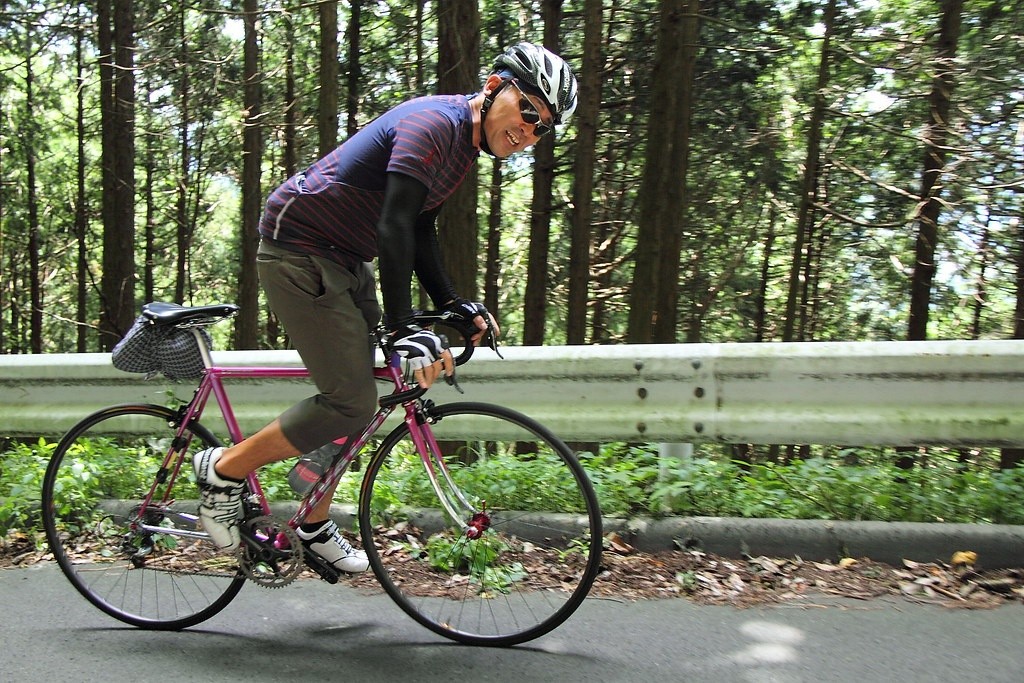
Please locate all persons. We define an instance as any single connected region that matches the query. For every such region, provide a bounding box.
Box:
[191,41,580,573]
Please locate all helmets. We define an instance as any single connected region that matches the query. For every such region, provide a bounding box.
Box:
[495,42,578,126]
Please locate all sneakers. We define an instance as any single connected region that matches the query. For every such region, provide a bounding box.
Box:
[290,520,373,576]
[192,446,243,551]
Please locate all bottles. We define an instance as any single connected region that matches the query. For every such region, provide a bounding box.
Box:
[287,437,348,494]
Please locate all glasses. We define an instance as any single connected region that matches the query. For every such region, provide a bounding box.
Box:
[511,79,552,138]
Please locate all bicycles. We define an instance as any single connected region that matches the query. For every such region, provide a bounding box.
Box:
[38,306,606,647]
